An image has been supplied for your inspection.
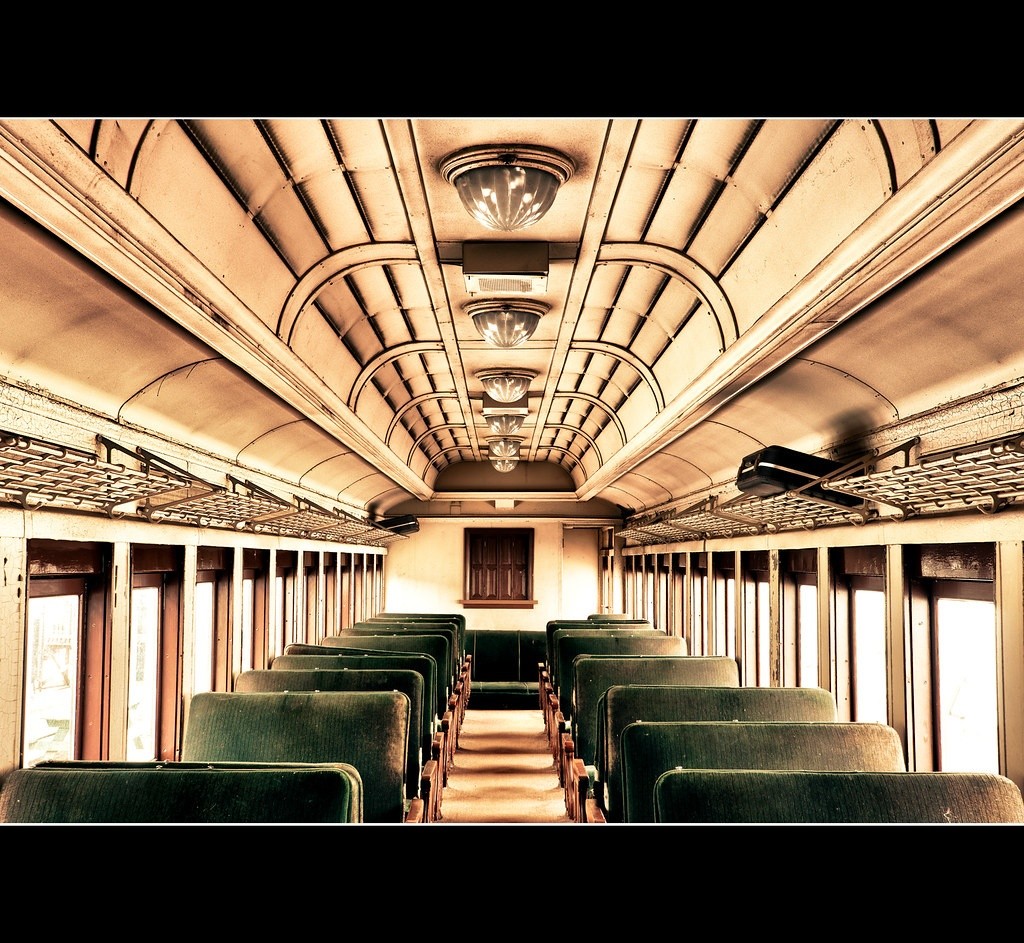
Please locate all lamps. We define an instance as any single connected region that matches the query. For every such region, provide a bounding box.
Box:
[490,460,519,473]
[483,414,527,435]
[475,368,538,403]
[462,299,554,348]
[484,435,526,457]
[438,143,579,232]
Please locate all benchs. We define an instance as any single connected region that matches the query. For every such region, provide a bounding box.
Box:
[466,630,547,709]
[0,613,473,831]
[538,619,1024,825]
